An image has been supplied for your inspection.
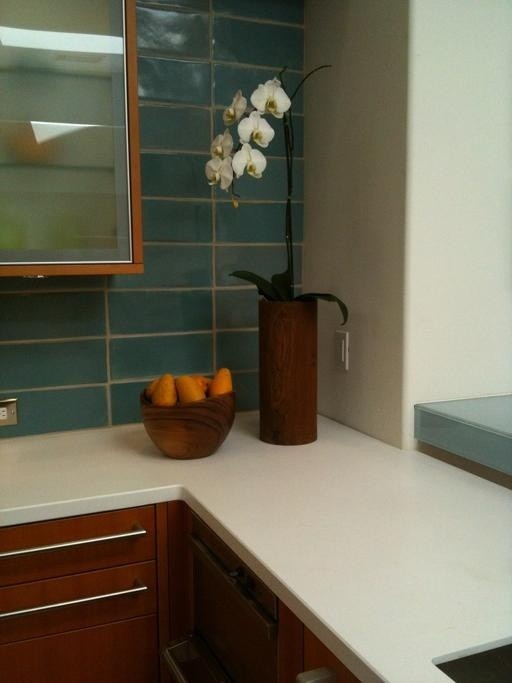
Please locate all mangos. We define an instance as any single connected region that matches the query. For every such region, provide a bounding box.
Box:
[146,367,233,406]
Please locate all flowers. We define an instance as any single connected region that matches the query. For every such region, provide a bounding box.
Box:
[201,63,351,327]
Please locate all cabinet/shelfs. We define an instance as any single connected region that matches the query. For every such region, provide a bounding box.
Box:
[1,0,147,277]
[163,501,368,682]
[0,497,171,683]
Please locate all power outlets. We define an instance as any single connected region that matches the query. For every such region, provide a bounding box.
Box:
[0,397,17,426]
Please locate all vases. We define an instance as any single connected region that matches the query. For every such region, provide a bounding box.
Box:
[256,296,321,446]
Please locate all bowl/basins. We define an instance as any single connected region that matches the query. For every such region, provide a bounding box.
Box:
[141,387,237,463]
